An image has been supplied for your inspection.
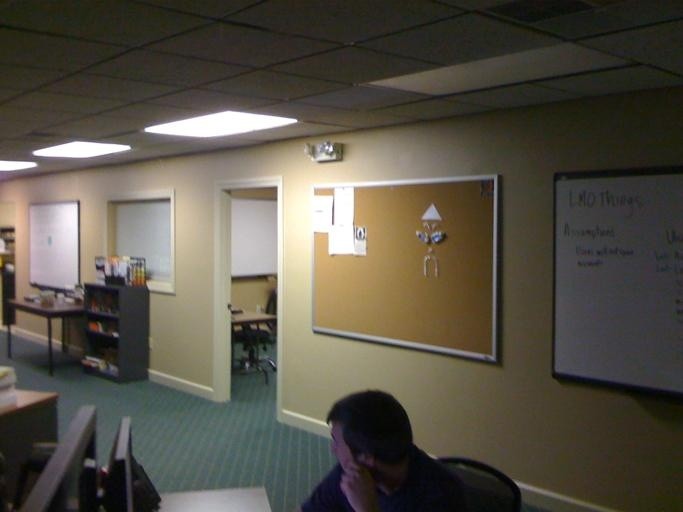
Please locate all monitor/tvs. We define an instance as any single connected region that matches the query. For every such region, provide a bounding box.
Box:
[108,416,135,511]
[18,404,96,512]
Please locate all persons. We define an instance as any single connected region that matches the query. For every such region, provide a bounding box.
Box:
[299,391,471,512]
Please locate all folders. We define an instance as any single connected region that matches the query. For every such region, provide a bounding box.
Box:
[104,256,146,288]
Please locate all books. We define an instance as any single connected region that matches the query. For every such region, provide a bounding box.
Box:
[82,354,117,376]
[103,255,145,287]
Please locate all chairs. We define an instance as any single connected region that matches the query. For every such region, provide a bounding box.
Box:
[436,456,522,512]
[235,289,277,375]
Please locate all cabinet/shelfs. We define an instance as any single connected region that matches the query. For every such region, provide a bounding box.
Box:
[80,280,150,384]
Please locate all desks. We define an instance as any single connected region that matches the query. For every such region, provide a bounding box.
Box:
[7,299,85,376]
[232,311,277,385]
[0,390,59,472]
